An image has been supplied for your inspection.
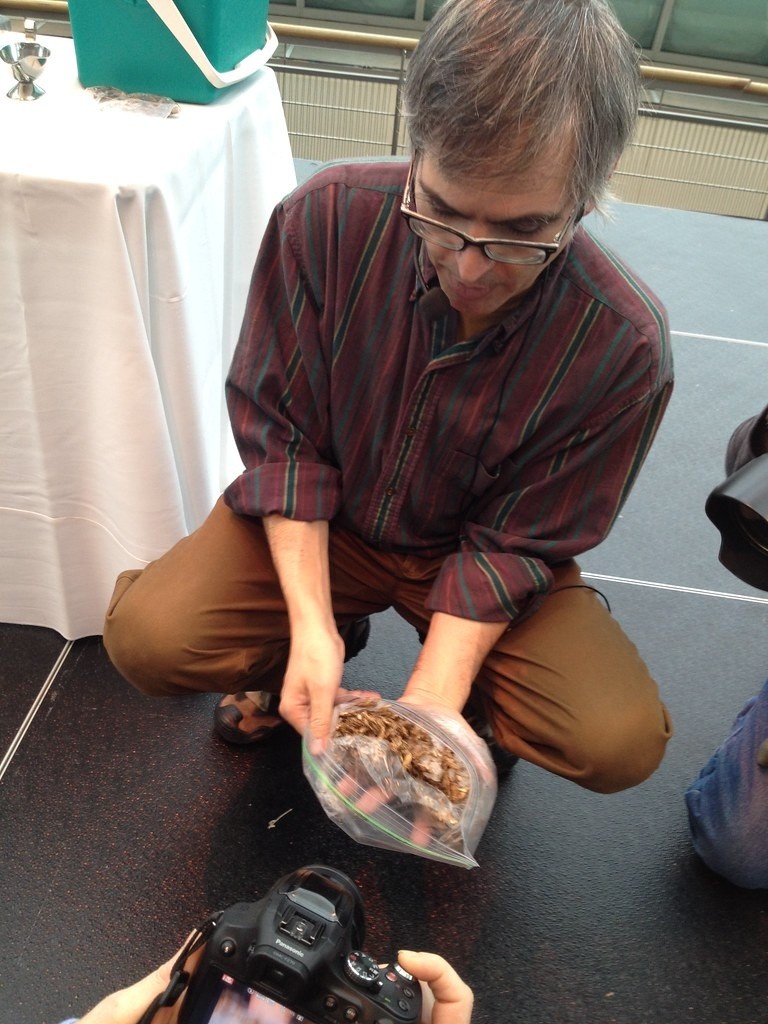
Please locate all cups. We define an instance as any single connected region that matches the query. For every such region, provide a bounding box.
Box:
[0,17,51,102]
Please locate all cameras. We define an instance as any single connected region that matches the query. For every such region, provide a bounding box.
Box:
[177,863,423,1024]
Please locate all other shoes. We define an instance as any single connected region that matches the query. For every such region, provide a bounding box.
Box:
[461,688,520,776]
[213,617,370,746]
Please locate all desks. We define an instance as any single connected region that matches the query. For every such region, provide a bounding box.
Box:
[0,30,298,640]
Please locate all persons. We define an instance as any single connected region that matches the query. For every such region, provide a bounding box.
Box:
[688,401,768,892]
[56,929,477,1024]
[103,0,705,854]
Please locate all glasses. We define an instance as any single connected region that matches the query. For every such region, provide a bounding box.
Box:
[400,147,586,267]
